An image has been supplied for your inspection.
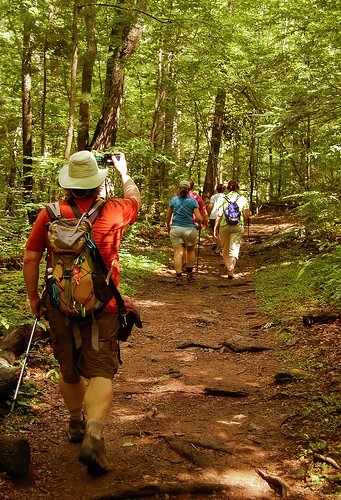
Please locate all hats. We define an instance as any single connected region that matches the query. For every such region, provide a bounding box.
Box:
[58,151,108,189]
[179,181,190,188]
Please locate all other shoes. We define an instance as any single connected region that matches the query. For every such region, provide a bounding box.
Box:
[186,271,193,281]
[174,276,183,286]
[78,434,110,473]
[211,244,217,250]
[65,414,87,444]
[227,271,236,279]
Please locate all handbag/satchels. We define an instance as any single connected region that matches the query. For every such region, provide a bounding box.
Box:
[116,295,142,343]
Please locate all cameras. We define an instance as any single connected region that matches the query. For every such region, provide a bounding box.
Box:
[96,154,120,165]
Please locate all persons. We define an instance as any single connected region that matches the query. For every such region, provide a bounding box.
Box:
[207,183,226,256]
[213,180,252,279]
[166,180,202,286]
[171,178,209,273]
[22,150,142,476]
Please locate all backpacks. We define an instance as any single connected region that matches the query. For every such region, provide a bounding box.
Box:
[223,195,241,225]
[45,198,114,321]
